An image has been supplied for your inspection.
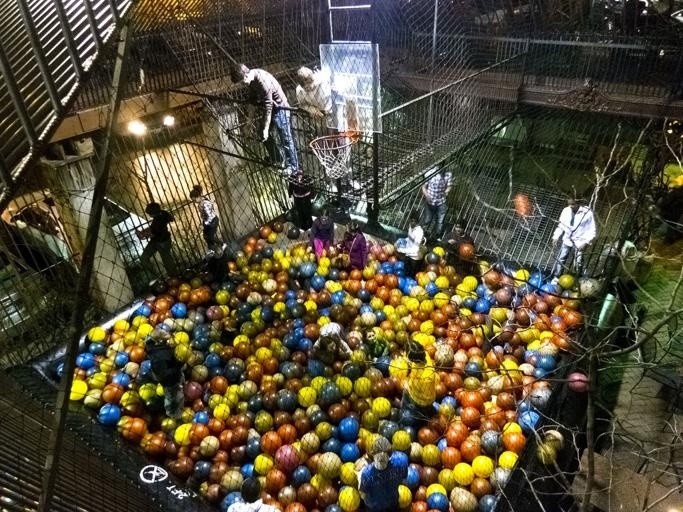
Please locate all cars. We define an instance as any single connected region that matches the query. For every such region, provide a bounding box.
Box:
[1,236,63,358]
[12,189,153,283]
[64,17,311,89]
[107,111,179,147]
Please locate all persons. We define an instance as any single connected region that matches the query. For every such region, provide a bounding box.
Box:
[145,328,184,416]
[356,437,407,512]
[393,210,426,273]
[135,202,181,287]
[339,219,367,271]
[547,194,599,275]
[292,61,365,194]
[228,62,302,179]
[189,185,227,258]
[227,478,281,512]
[402,340,437,411]
[314,333,353,365]
[440,218,475,251]
[283,175,315,231]
[420,160,454,238]
[306,205,336,263]
[200,250,230,285]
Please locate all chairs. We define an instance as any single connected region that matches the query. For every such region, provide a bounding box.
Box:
[657,310,682,365]
[594,432,649,476]
[655,432,683,494]
[629,337,682,411]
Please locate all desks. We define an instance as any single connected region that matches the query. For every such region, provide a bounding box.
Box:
[570,447,683,512]
